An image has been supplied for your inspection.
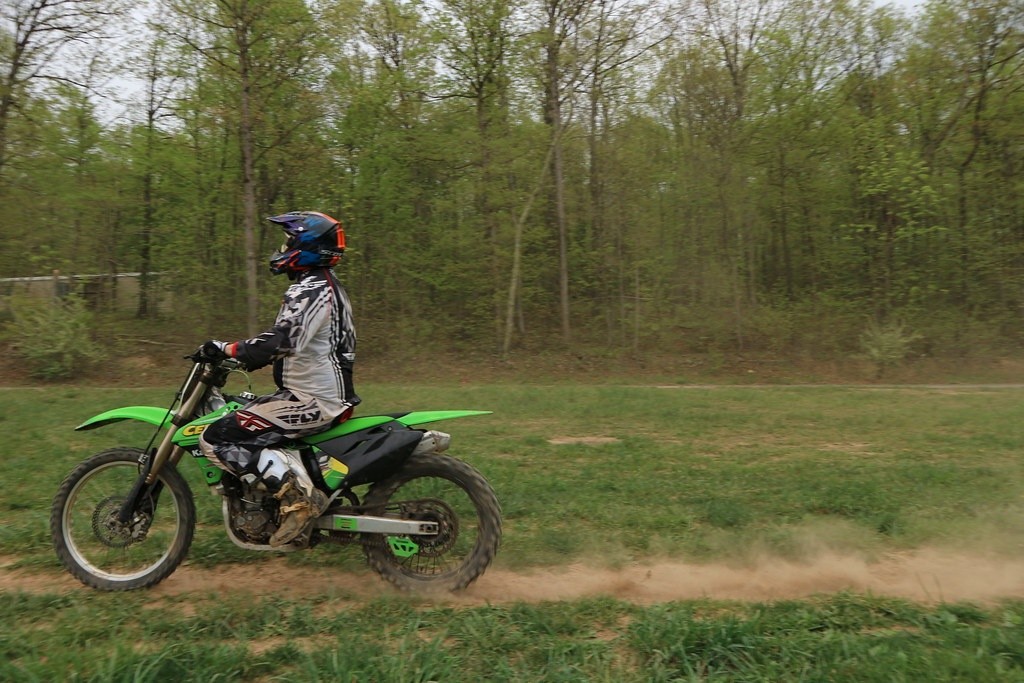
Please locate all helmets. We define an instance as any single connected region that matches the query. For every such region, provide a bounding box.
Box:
[268,211,346,277]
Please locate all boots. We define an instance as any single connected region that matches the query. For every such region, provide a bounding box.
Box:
[269,470,330,549]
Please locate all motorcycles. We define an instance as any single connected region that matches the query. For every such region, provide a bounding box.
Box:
[50,343,502,596]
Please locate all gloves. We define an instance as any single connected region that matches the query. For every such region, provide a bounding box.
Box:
[202,339,233,361]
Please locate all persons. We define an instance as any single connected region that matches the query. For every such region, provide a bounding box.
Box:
[199,210,359,547]
